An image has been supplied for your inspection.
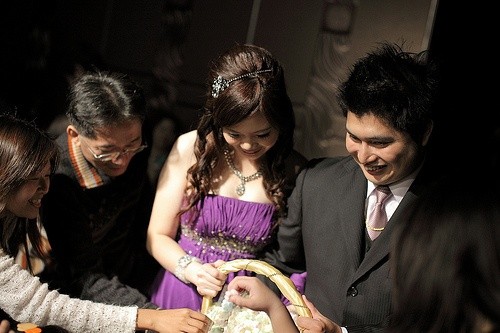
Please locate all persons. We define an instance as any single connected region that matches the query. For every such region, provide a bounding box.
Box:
[41,72,159,291]
[256,42,457,333]
[227,276,301,333]
[147,80,192,184]
[147,45,308,313]
[0,114,214,333]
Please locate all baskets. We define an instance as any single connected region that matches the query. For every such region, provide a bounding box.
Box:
[201,258,313,333]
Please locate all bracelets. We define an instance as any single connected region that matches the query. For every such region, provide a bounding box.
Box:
[175,255,205,284]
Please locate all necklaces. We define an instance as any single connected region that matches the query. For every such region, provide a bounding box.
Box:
[223,144,264,196]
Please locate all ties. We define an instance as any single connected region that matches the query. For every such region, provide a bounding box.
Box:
[365,185,393,240]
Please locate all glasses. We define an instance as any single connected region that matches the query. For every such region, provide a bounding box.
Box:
[86,140,148,161]
